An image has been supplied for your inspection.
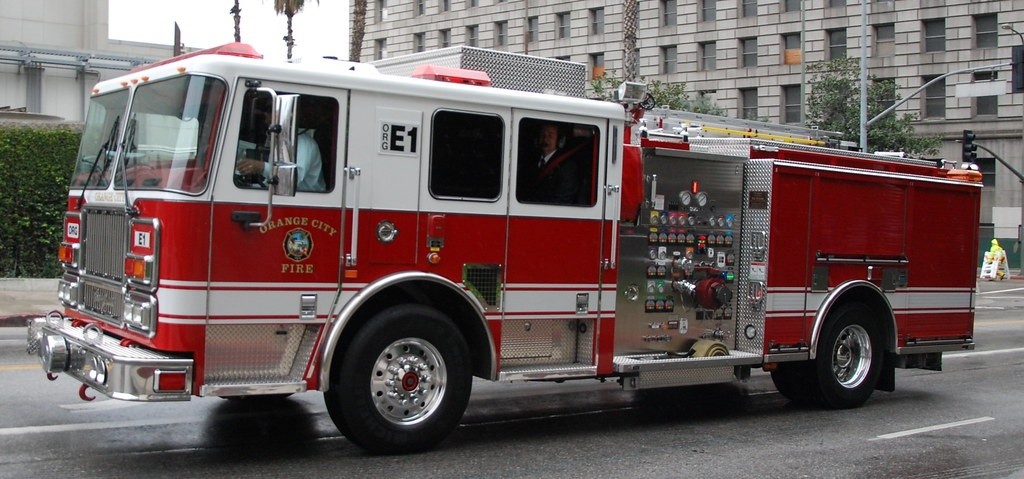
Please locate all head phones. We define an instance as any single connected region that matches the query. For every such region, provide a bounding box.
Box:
[533,123,566,150]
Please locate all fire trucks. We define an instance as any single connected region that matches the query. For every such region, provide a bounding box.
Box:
[25,41,985,453]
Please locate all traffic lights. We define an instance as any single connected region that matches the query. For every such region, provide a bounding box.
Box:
[962,129,977,161]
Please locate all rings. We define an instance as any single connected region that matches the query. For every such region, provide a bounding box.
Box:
[248,165,252,168]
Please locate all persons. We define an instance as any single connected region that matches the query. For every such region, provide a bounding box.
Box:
[236,99,326,193]
[989,239,1005,280]
[527,125,579,203]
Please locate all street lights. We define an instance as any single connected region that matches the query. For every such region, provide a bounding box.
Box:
[1001,24,1024,46]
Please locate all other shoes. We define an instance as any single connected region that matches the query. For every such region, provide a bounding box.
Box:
[1001,274,1005,280]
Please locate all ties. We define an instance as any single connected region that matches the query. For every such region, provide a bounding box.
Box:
[540,155,546,168]
